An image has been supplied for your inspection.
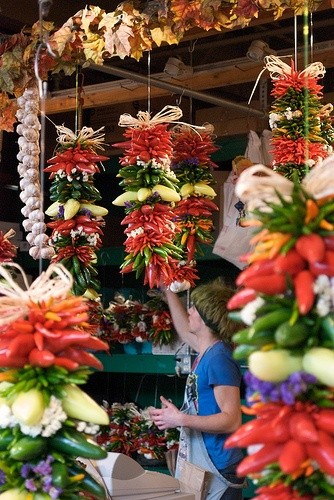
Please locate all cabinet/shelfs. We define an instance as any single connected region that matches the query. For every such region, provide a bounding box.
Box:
[14,233,257,500]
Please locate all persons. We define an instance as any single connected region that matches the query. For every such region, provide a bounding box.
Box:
[150,275,247,500]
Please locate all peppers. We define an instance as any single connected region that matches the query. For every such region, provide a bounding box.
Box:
[0,58,334,500]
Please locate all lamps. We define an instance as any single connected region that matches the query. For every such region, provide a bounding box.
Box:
[165,57,193,78]
[247,40,278,61]
